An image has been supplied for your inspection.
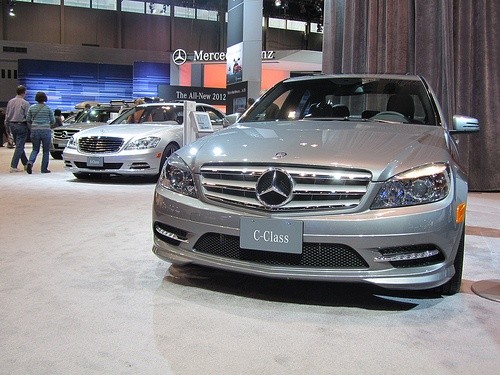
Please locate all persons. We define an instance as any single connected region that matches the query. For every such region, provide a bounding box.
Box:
[4,85,30,174]
[26,92,56,174]
[0,98,152,149]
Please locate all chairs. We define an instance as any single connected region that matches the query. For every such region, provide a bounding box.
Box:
[309,101,349,120]
[386,94,416,124]
[153,110,166,122]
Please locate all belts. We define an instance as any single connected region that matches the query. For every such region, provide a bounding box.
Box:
[11,122,26,124]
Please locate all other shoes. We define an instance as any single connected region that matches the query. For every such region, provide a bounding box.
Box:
[24,165,27,170]
[41,169,51,173]
[27,164,32,174]
[10,166,23,173]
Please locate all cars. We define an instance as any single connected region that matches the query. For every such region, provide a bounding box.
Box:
[151,71,480,296]
[48,100,137,160]
[61,102,227,183]
